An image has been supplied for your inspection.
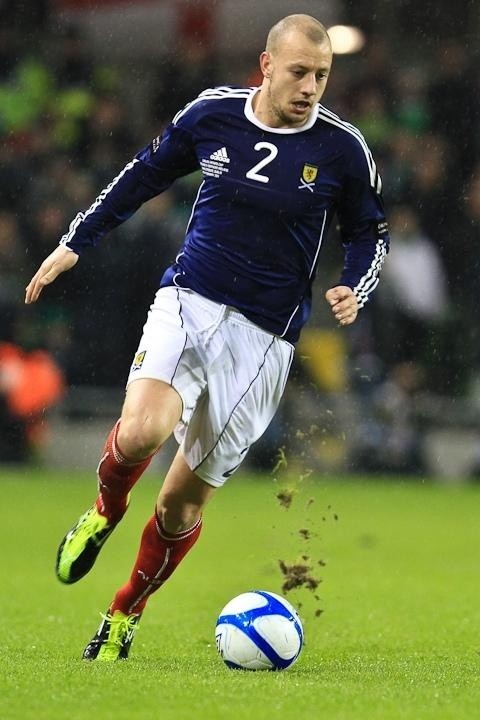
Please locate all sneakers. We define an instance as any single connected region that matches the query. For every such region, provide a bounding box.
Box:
[53,490,132,586]
[81,598,142,664]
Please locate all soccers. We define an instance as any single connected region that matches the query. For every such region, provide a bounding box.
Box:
[215,591,303,671]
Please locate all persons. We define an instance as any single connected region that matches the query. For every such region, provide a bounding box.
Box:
[20,12,396,663]
[1,0,479,489]
